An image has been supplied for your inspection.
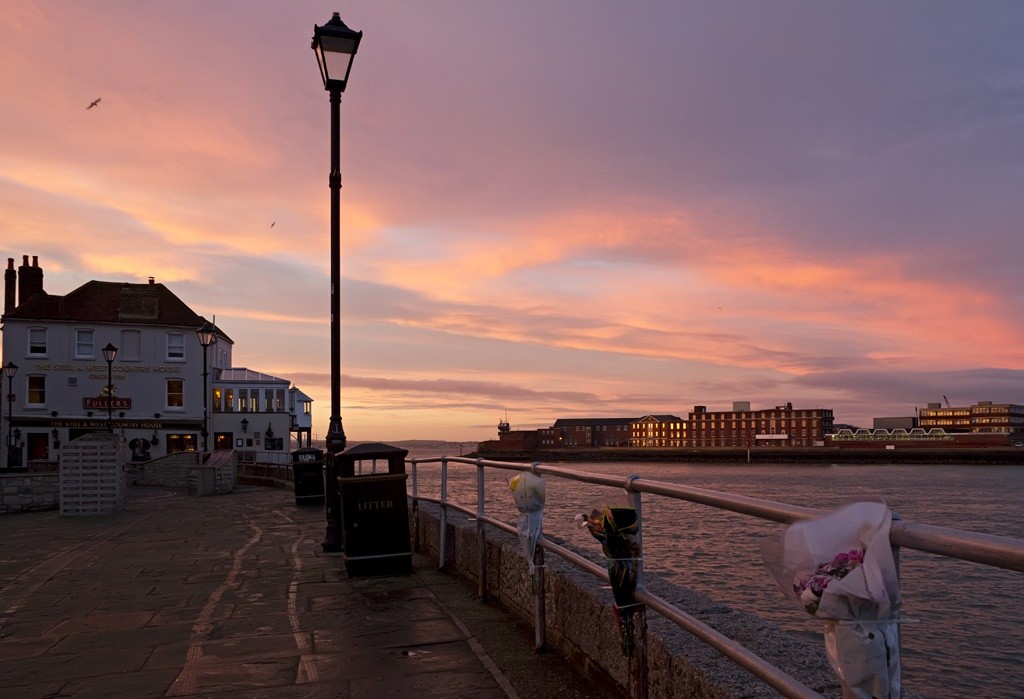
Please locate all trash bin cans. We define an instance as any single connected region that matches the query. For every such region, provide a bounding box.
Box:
[334,441,413,579]
[291,446,325,507]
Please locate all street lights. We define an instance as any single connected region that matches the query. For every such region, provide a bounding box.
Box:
[197,322,215,463]
[101,343,118,434]
[3,361,18,470]
[309,10,365,553]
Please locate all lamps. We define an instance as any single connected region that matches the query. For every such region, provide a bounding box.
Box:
[14,411,248,446]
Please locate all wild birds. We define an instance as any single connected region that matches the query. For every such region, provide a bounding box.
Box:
[86,98,102,110]
[271,221,276,229]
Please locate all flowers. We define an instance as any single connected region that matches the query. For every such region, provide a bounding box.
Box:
[509,462,546,577]
[578,506,643,656]
[759,503,906,699]
